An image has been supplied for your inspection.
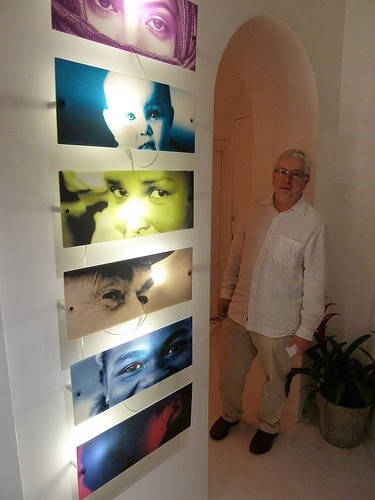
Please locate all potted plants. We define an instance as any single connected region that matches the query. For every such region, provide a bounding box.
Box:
[285,331,375,449]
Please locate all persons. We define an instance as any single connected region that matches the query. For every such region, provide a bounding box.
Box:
[63,249,174,338]
[104,169,194,238]
[210,148,326,456]
[87,316,193,417]
[103,70,179,151]
[137,383,192,461]
[52,0,199,71]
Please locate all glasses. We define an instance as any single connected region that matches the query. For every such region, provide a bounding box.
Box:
[278,169,306,179]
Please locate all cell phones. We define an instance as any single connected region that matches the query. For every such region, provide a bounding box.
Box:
[286,343,298,358]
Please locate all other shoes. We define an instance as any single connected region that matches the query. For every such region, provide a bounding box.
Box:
[210,416,239,440]
[249,429,278,454]
